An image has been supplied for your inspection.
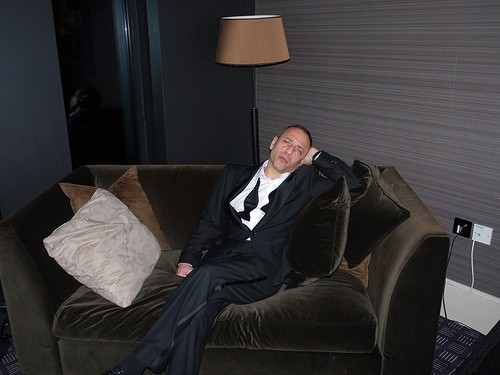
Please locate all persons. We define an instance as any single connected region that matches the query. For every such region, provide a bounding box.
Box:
[100,124,360,375]
[67,85,105,132]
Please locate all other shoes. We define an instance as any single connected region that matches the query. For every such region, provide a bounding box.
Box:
[100,356,146,375]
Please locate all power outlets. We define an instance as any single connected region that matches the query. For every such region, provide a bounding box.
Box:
[452,217,472,238]
[472,223,494,246]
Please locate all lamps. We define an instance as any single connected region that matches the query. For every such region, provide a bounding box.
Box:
[214,15,290,164]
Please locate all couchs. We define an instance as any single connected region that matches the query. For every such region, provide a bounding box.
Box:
[0,159,451,375]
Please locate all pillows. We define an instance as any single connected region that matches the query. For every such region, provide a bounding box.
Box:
[60,165,171,253]
[286,175,352,280]
[344,159,410,269]
[43,189,162,309]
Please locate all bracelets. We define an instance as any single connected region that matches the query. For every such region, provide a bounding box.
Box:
[312,151,322,162]
